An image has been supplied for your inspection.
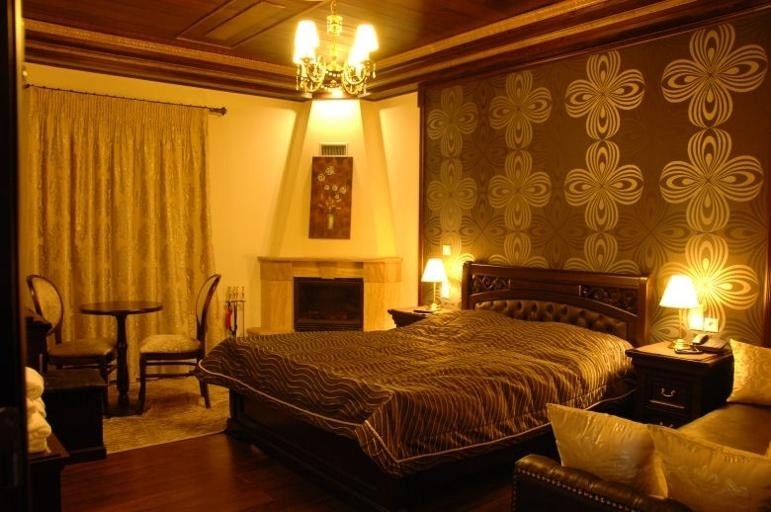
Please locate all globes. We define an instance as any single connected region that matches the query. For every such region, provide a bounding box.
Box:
[23,275,64,337]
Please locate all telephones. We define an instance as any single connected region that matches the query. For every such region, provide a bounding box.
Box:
[689,333,728,352]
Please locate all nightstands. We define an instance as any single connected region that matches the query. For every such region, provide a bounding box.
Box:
[624,341,734,431]
[385,306,453,327]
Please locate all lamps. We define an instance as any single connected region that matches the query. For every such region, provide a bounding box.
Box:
[292,1,379,100]
[414,258,447,313]
[658,275,698,351]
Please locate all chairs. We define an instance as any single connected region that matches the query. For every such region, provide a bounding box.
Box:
[134,274,221,415]
[27,275,120,419]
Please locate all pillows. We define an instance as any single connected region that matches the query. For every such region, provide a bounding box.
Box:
[645,424,771,512]
[727,336,771,408]
[544,402,668,501]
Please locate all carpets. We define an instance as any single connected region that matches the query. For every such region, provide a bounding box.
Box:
[33,359,231,455]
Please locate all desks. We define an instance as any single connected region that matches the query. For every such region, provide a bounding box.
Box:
[34,367,106,466]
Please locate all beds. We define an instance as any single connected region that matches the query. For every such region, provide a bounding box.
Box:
[196,259,652,512]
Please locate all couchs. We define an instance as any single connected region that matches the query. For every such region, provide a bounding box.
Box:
[512,339,771,512]
